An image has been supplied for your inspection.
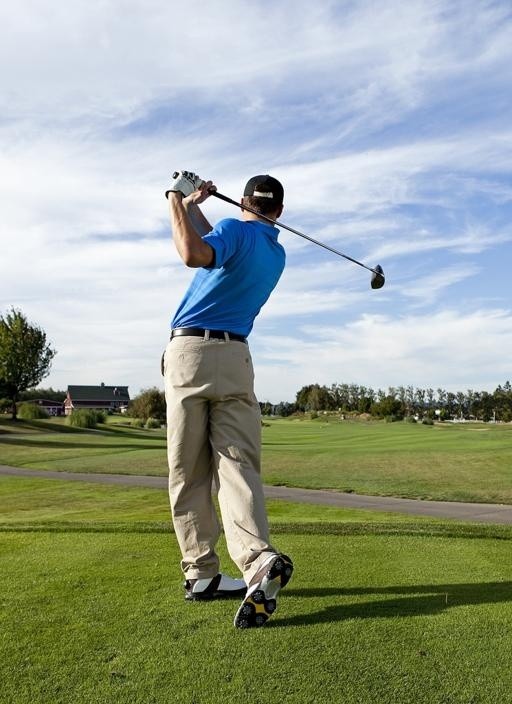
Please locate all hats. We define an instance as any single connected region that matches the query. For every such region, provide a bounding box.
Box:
[244,175,284,203]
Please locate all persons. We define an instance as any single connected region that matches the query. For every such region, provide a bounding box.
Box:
[160,170,293,628]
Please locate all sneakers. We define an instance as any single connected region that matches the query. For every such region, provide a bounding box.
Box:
[184,573,247,600]
[234,554,293,628]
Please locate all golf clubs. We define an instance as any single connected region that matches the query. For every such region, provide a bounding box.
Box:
[172,172,384,290]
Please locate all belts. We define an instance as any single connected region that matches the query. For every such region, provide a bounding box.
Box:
[171,328,248,344]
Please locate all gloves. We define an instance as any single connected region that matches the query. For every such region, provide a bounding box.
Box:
[165,170,203,199]
